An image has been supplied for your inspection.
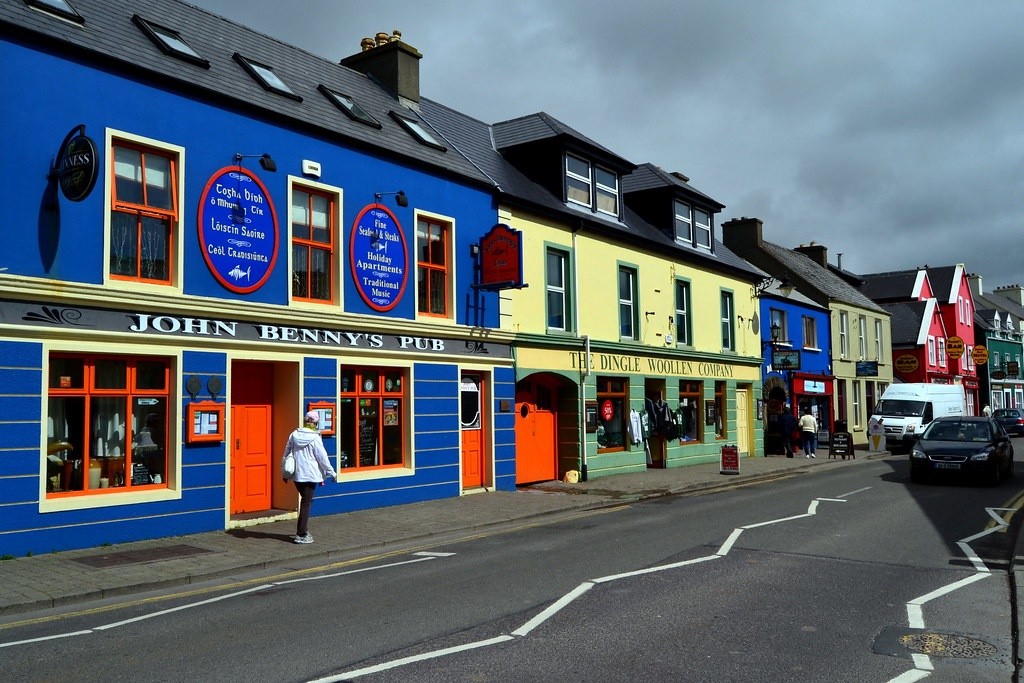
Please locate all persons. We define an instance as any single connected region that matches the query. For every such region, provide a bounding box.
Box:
[281,410,337,543]
[982,404,992,417]
[777,407,818,458]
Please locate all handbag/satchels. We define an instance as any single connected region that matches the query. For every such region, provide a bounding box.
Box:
[281,436,295,479]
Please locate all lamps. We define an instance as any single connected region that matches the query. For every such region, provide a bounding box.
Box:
[375,190,410,207]
[234,153,276,172]
[752,270,797,302]
[761,322,781,352]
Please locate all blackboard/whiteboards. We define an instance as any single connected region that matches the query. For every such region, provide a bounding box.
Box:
[829,431,855,456]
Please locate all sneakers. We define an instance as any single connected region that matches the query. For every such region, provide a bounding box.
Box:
[306,531,313,539]
[294,535,314,544]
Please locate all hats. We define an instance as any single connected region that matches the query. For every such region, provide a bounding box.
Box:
[305,411,319,423]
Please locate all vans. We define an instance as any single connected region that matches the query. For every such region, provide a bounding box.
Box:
[866,382,968,454]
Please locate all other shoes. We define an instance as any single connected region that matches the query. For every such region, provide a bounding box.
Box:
[811,453,816,458]
[806,455,809,459]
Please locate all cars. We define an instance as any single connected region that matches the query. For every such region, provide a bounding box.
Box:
[992,408,1024,437]
[909,416,1013,489]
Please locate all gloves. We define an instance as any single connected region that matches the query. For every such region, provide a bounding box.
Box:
[332,472,337,481]
